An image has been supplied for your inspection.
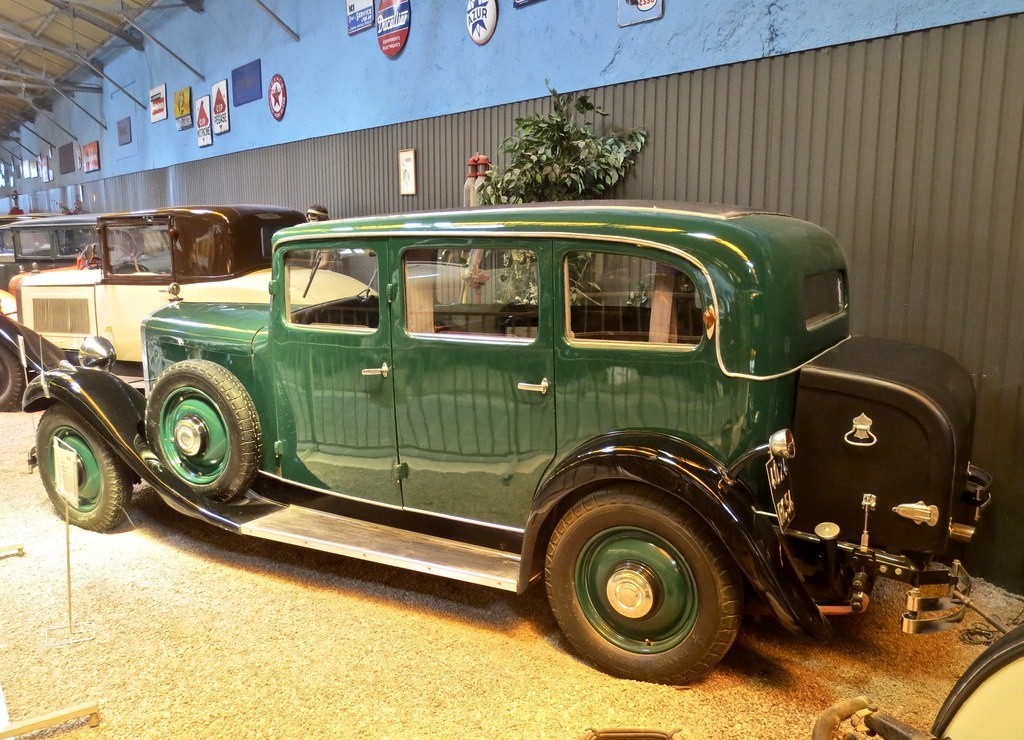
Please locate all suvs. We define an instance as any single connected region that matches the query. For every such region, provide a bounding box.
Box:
[21,197,998,685]
[0,206,311,413]
